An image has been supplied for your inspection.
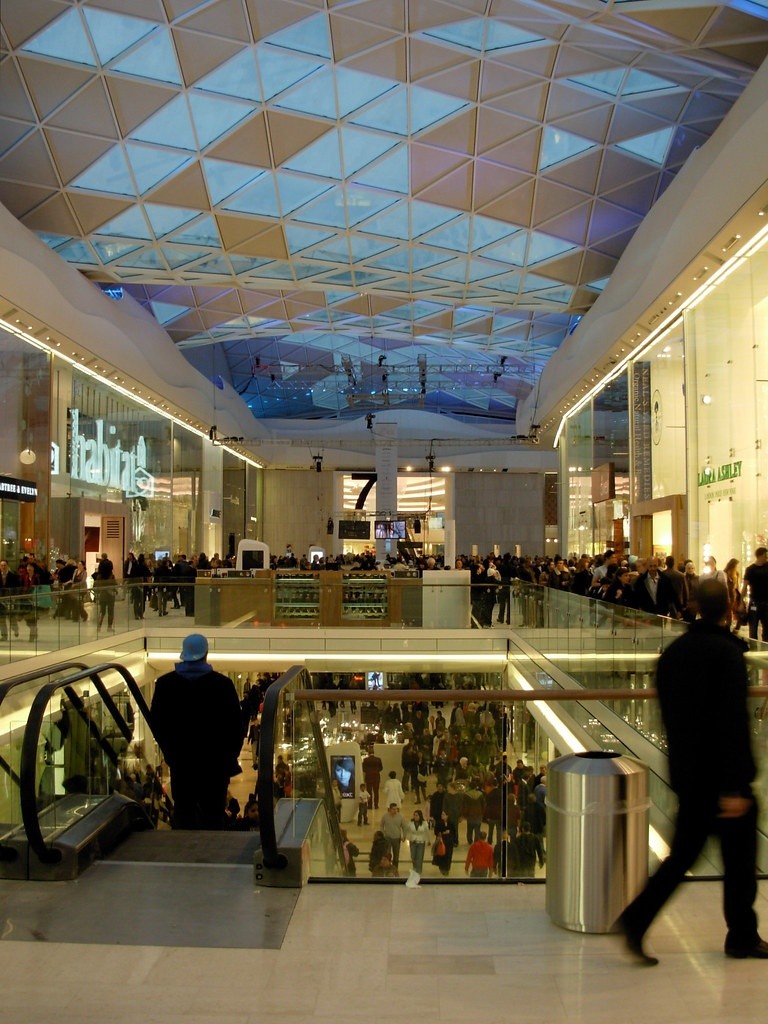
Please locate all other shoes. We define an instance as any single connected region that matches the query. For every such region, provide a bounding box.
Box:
[497,619,504,623]
[83,613,88,621]
[506,620,510,625]
[724,941,768,958]
[0,635,8,641]
[15,630,19,637]
[107,628,114,632]
[608,920,659,966]
[159,611,168,617]
[135,616,144,620]
[97,624,101,632]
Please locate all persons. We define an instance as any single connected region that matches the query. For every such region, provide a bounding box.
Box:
[0,543,768,642]
[150,635,242,830]
[610,579,768,965]
[132,673,548,875]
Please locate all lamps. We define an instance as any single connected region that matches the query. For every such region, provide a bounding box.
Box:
[209,353,543,472]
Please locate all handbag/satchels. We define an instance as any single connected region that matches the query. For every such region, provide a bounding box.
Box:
[418,774,427,782]
[39,767,55,810]
[107,589,116,596]
[431,831,446,856]
[150,595,158,608]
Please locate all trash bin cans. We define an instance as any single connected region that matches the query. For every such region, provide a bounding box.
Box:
[547,752,651,935]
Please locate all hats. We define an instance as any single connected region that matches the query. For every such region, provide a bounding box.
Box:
[180,634,208,661]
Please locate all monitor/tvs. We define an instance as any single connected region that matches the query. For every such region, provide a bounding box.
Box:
[242,551,263,571]
[375,520,406,539]
[310,551,323,564]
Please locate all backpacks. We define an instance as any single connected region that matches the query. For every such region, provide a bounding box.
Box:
[343,841,351,864]
